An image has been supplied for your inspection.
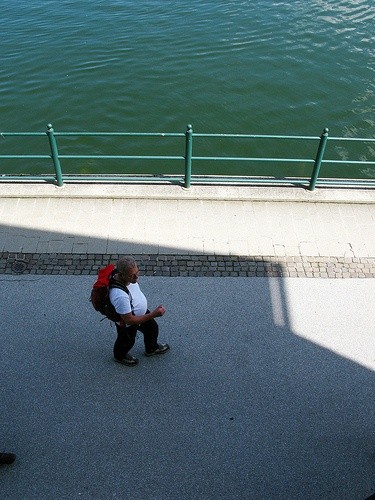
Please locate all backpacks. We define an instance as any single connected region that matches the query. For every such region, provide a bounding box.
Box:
[89,263,135,323]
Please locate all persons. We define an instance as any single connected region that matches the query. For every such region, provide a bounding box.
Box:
[107,255,170,367]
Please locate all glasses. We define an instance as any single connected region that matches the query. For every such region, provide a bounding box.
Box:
[127,270,140,280]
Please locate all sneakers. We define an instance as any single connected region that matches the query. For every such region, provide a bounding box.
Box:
[145,343,169,356]
[113,355,138,366]
